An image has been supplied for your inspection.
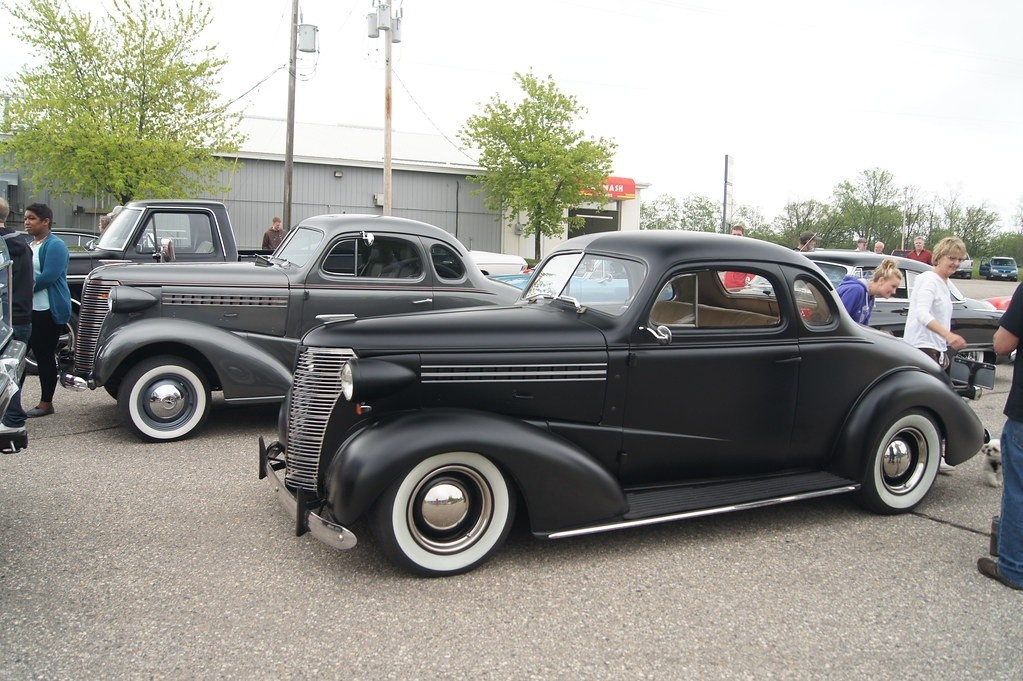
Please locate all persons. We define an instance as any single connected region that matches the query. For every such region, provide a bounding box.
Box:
[793,231,816,252]
[0,197,34,432]
[834,260,902,326]
[874,241,885,255]
[903,236,968,368]
[22,204,71,419]
[906,236,932,264]
[976,279,1023,590]
[261,217,288,250]
[856,238,868,251]
[98,205,124,233]
[723,226,757,288]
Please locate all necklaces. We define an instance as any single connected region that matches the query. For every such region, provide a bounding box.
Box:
[30,236,47,245]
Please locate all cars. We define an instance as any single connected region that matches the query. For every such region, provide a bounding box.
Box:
[62,215,525,443]
[259,230,991,576]
[731,249,1017,367]
[1,227,179,372]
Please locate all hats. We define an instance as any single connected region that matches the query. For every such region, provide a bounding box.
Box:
[800,231,822,243]
[858,238,867,243]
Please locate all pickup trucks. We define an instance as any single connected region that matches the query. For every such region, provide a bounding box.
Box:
[61,199,367,340]
[890,249,914,258]
[949,253,973,279]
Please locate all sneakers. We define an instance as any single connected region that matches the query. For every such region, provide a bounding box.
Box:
[938,457,955,471]
[977,558,1023,590]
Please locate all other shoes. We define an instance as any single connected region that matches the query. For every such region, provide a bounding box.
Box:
[26,402,54,418]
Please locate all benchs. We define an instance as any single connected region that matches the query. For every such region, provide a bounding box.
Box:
[371,261,418,279]
[653,300,779,328]
[196,240,213,254]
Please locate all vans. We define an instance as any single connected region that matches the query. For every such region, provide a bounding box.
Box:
[978,255,1018,281]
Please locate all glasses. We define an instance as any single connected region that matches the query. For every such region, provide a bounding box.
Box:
[946,254,964,263]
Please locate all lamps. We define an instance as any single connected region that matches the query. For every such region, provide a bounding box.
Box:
[334,170,343,176]
